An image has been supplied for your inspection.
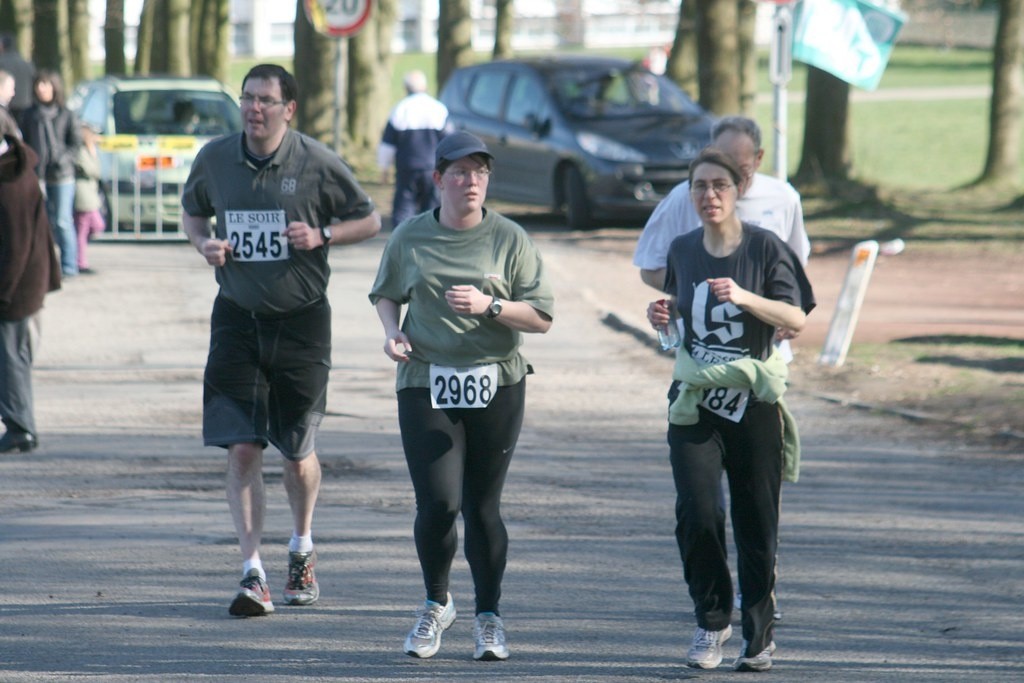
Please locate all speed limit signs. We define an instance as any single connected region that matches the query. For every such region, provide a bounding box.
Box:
[303,0,375,36]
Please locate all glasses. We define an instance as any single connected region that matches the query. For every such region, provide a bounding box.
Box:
[444,169,491,181]
[689,182,737,195]
[238,96,285,109]
[743,163,757,176]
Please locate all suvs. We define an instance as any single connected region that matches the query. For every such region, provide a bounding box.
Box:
[65,76,242,231]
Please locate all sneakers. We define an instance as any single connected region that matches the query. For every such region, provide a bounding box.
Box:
[732,639,776,672]
[403,592,456,659]
[473,611,509,660]
[229,568,274,616]
[283,546,319,604]
[686,625,732,669]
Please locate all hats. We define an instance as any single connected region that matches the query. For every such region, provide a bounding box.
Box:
[434,131,495,168]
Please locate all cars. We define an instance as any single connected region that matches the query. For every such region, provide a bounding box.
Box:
[438,55,717,227]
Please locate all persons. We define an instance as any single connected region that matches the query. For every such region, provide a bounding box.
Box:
[0,108,64,450]
[181,63,381,616]
[378,71,454,226]
[647,152,817,673]
[368,131,554,660]
[0,70,104,276]
[634,115,811,618]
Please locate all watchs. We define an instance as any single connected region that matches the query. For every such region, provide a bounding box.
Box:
[319,226,332,248]
[487,296,502,318]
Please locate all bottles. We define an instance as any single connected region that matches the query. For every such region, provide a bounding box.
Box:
[654,298,680,351]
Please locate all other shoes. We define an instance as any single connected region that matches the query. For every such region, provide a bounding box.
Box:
[0,429,36,455]
